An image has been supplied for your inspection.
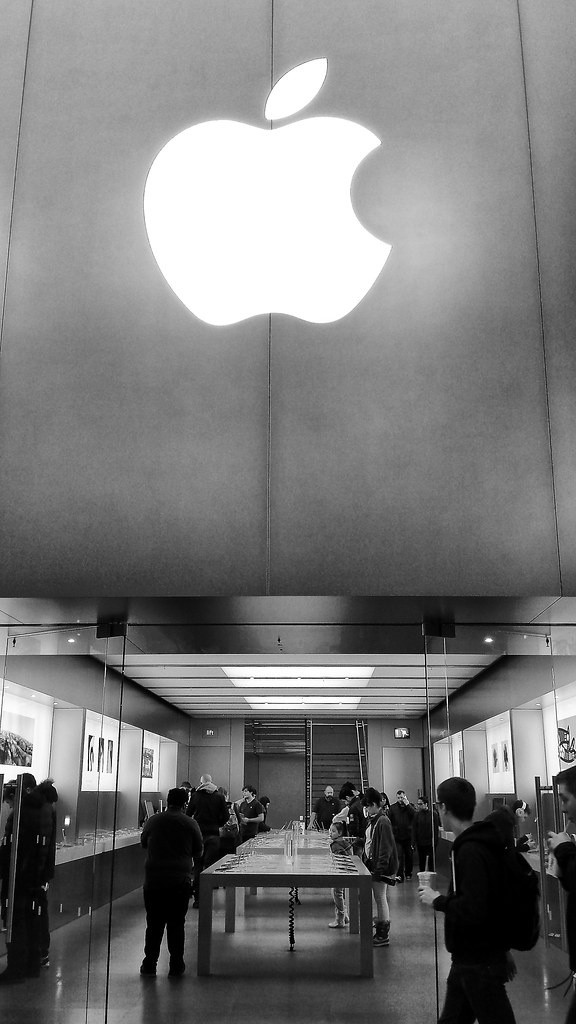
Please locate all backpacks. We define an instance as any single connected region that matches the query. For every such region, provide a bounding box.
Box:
[487,835,540,951]
[219,804,239,838]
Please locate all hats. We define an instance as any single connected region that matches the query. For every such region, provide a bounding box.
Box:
[180,782,190,788]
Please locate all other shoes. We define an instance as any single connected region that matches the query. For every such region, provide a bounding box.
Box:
[41,955,49,966]
[140,965,156,977]
[328,916,350,927]
[168,969,184,977]
[0,969,25,983]
[373,936,389,946]
[193,902,199,909]
[23,967,40,977]
[407,874,411,880]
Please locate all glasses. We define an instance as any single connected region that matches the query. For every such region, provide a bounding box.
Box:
[435,802,445,809]
[417,803,426,806]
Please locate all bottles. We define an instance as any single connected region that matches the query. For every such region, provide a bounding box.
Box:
[299,816,306,834]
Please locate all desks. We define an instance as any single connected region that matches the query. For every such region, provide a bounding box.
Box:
[196,828,373,980]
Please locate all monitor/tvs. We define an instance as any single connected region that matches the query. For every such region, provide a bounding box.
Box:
[492,797,506,812]
[144,800,163,820]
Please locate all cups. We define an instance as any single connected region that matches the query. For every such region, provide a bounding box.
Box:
[547,851,558,878]
[417,872,437,891]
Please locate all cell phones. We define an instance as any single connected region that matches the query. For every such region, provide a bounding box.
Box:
[215,854,250,871]
[334,854,358,871]
[87,735,113,774]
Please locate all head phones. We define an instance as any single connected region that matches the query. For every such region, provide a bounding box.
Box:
[515,801,527,816]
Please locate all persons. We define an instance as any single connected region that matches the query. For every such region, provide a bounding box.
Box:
[179,774,230,908]
[482,800,535,852]
[410,797,439,871]
[418,777,517,1024]
[140,789,204,977]
[0,773,58,983]
[331,782,371,860]
[235,785,273,843]
[329,821,351,928]
[308,786,341,830]
[361,788,399,947]
[546,765,576,1024]
[381,791,415,883]
[217,786,242,889]
